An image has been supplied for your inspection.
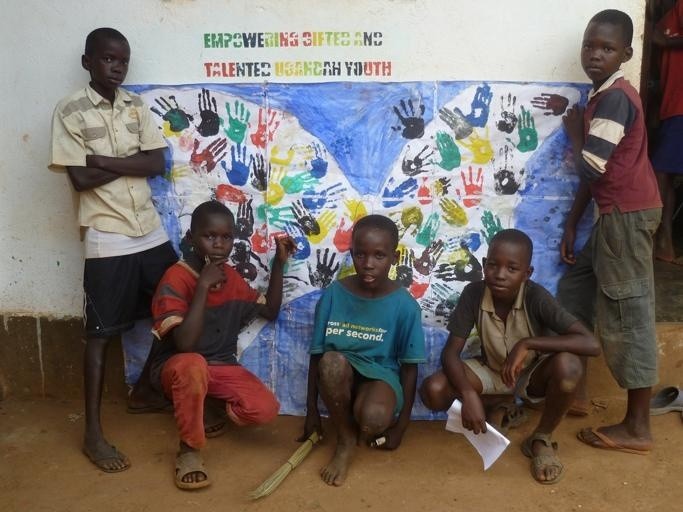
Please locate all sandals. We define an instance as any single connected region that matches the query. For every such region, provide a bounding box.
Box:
[520,432,566,485]
[485,401,527,436]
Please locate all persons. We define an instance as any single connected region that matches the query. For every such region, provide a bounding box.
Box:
[416,226,604,485]
[292,213,430,487]
[641,1,682,266]
[47,27,182,473]
[552,6,664,455]
[151,199,299,491]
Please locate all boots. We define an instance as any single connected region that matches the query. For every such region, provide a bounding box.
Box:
[172,448,210,489]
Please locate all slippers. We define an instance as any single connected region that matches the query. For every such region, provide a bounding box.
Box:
[125,399,174,415]
[650,386,682,416]
[203,411,227,438]
[577,425,650,455]
[82,441,130,472]
